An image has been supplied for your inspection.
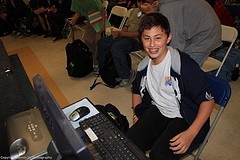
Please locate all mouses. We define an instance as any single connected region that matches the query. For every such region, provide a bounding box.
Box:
[69,106,90,122]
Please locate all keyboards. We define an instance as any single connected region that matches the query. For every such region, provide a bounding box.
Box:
[79,113,150,160]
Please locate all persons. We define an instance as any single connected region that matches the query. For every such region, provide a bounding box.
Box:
[128,11,215,160]
[0,0,223,88]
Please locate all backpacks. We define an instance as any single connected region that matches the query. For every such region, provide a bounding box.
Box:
[65,39,93,78]
[96,37,122,88]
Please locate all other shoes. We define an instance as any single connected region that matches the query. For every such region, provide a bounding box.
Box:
[115,69,136,87]
[0,29,61,41]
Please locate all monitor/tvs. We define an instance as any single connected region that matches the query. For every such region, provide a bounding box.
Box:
[33,74,94,160]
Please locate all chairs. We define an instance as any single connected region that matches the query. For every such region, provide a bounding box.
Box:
[146,71,231,160]
[199,25,238,76]
[62,0,127,42]
